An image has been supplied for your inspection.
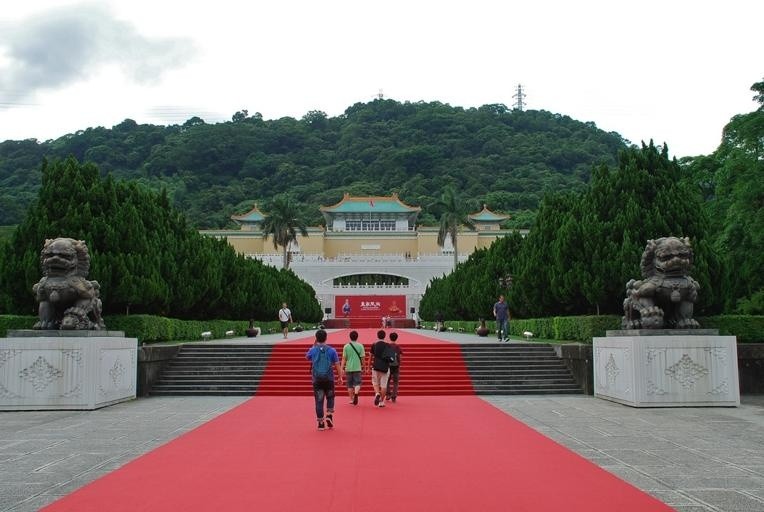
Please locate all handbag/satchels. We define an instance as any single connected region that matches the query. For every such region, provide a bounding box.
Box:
[374,346,398,372]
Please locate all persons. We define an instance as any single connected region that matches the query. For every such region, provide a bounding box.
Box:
[279,302,293,338]
[381,314,392,329]
[367,330,403,407]
[305,330,343,430]
[340,330,367,405]
[493,295,510,342]
[433,310,443,335]
[342,298,352,317]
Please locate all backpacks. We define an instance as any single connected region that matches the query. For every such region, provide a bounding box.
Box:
[313,345,330,378]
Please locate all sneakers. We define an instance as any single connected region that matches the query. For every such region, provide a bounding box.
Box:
[374,393,396,407]
[499,338,509,342]
[318,415,333,430]
[350,394,358,405]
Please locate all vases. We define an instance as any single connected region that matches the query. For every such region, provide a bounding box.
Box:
[245,318,259,337]
[434,320,447,332]
[415,321,422,329]
[295,319,304,332]
[320,321,325,329]
[476,318,490,337]
